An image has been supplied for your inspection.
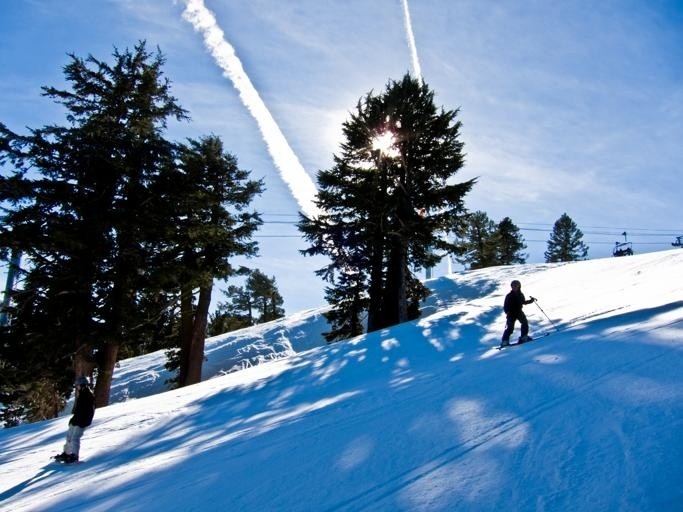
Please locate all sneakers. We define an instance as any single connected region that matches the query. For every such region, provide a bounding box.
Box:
[518,336,532,344]
[501,337,510,347]
[54,452,78,464]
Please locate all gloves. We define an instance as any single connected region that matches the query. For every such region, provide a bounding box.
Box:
[531,298,534,302]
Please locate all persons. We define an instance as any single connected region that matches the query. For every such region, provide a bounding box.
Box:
[502,280,534,346]
[54,376,95,463]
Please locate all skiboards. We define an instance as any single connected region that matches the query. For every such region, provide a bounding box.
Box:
[50,457,84,467]
[492,333,549,351]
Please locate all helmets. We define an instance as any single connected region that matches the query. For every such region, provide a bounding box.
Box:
[74,376,90,386]
[511,280,521,288]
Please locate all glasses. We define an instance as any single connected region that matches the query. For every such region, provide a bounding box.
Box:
[72,384,78,388]
[511,286,519,288]
